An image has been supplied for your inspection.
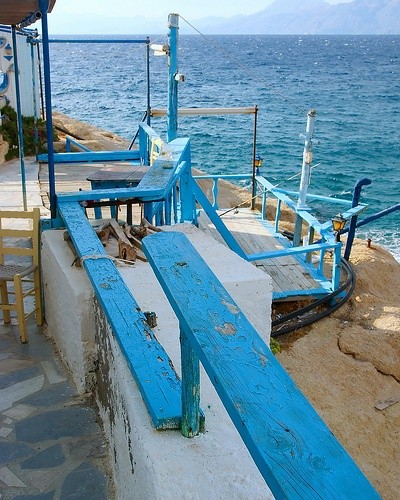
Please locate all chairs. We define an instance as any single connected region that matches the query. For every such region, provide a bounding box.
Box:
[0,208,42,344]
